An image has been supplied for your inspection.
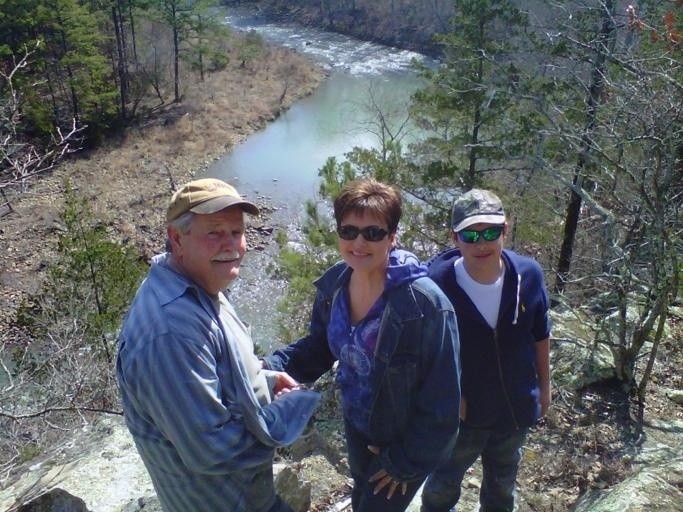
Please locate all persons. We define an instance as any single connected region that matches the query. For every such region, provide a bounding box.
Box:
[116,178,322,512]
[258,179,461,512]
[420,189,551,512]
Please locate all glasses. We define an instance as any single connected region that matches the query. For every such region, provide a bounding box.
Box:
[337,224,386,241]
[458,226,502,243]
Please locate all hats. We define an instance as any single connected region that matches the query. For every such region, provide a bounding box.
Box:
[165,178,259,221]
[450,188,505,233]
